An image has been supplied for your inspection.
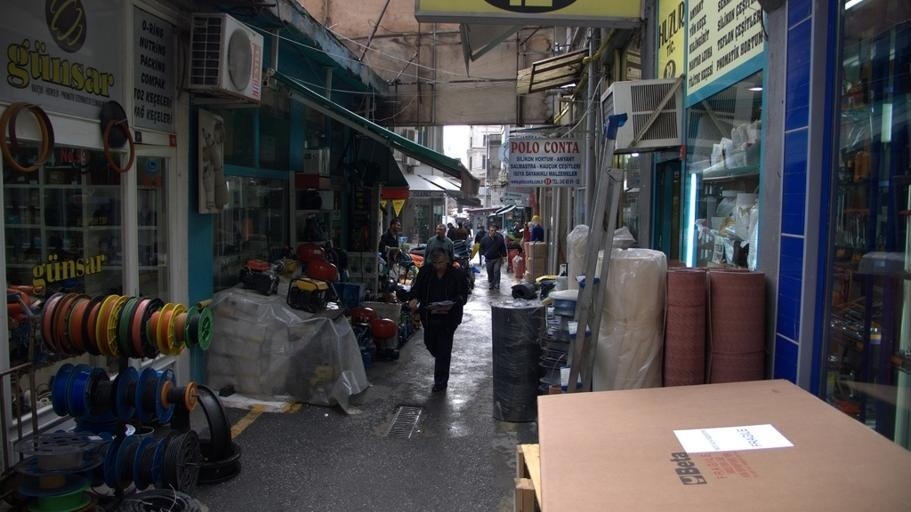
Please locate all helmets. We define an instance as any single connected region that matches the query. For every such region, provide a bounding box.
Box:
[532,216,540,221]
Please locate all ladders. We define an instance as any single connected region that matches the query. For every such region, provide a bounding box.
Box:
[567,110,630,393]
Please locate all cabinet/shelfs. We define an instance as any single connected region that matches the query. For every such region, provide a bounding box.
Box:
[1,139,164,303]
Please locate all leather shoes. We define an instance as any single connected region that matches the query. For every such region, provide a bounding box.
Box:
[432,384,447,391]
[489,284,499,289]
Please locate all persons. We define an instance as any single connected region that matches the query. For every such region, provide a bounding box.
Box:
[377,218,401,262]
[408,248,470,393]
[423,218,506,290]
[531,216,544,242]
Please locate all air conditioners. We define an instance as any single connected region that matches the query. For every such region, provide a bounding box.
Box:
[186,12,264,104]
[599,79,681,154]
